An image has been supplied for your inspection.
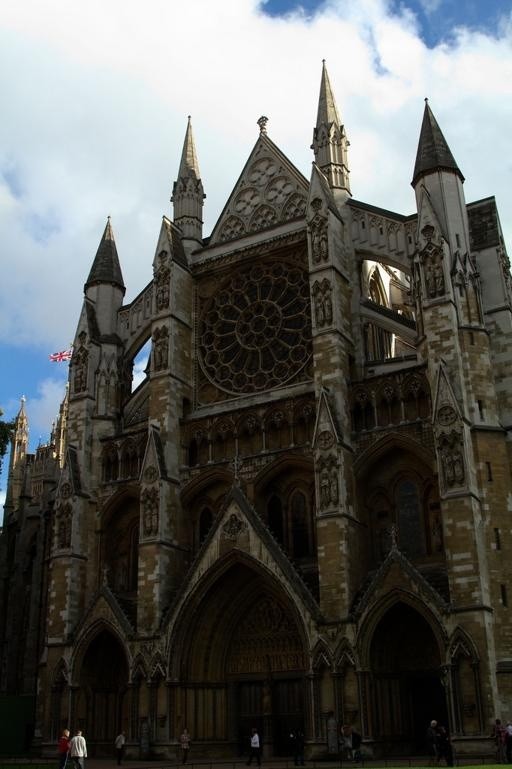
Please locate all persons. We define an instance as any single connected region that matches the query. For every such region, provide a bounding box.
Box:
[56,729,71,768]
[504,721,512,762]
[245,728,261,766]
[491,719,508,764]
[114,730,126,765]
[426,719,439,766]
[293,731,306,766]
[179,727,191,763]
[68,729,88,769]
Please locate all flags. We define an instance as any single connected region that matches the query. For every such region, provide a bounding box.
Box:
[49,350,71,363]
